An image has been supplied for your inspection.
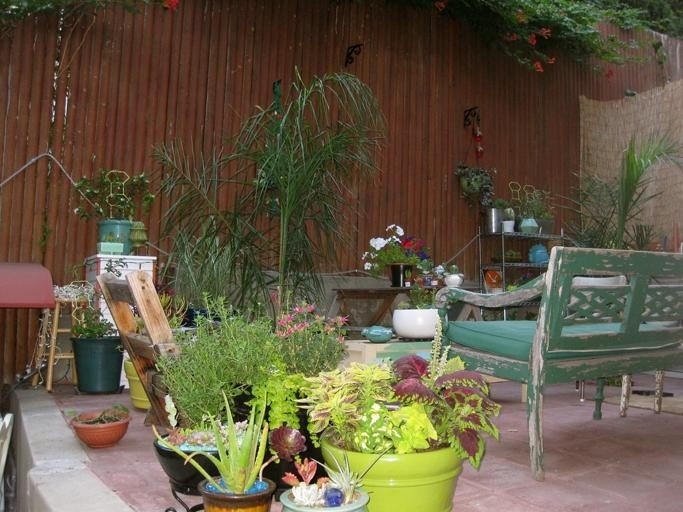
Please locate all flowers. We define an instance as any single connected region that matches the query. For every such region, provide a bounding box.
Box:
[357,221,431,276]
[268,298,357,351]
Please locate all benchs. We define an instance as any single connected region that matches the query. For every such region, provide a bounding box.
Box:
[427,240,682,484]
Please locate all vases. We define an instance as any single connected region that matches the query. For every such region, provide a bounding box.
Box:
[386,257,416,290]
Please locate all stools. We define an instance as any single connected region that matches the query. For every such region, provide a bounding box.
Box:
[25,294,93,398]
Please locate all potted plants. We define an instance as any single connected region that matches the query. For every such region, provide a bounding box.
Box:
[295,314,502,511]
[67,303,127,398]
[150,385,283,511]
[441,263,467,289]
[453,160,567,241]
[279,440,395,511]
[63,403,134,450]
[391,282,444,342]
[151,292,285,495]
[123,293,189,410]
[71,164,155,258]
